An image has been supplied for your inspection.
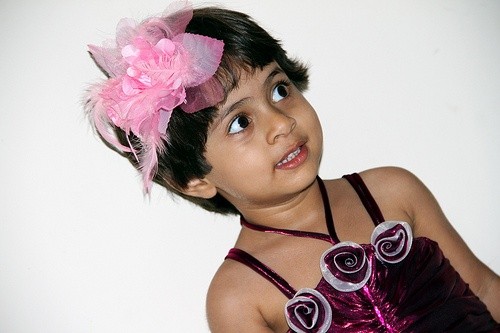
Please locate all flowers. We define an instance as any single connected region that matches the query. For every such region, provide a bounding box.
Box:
[82,1,225,199]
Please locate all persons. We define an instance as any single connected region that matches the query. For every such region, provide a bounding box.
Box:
[84,7,500,333]
